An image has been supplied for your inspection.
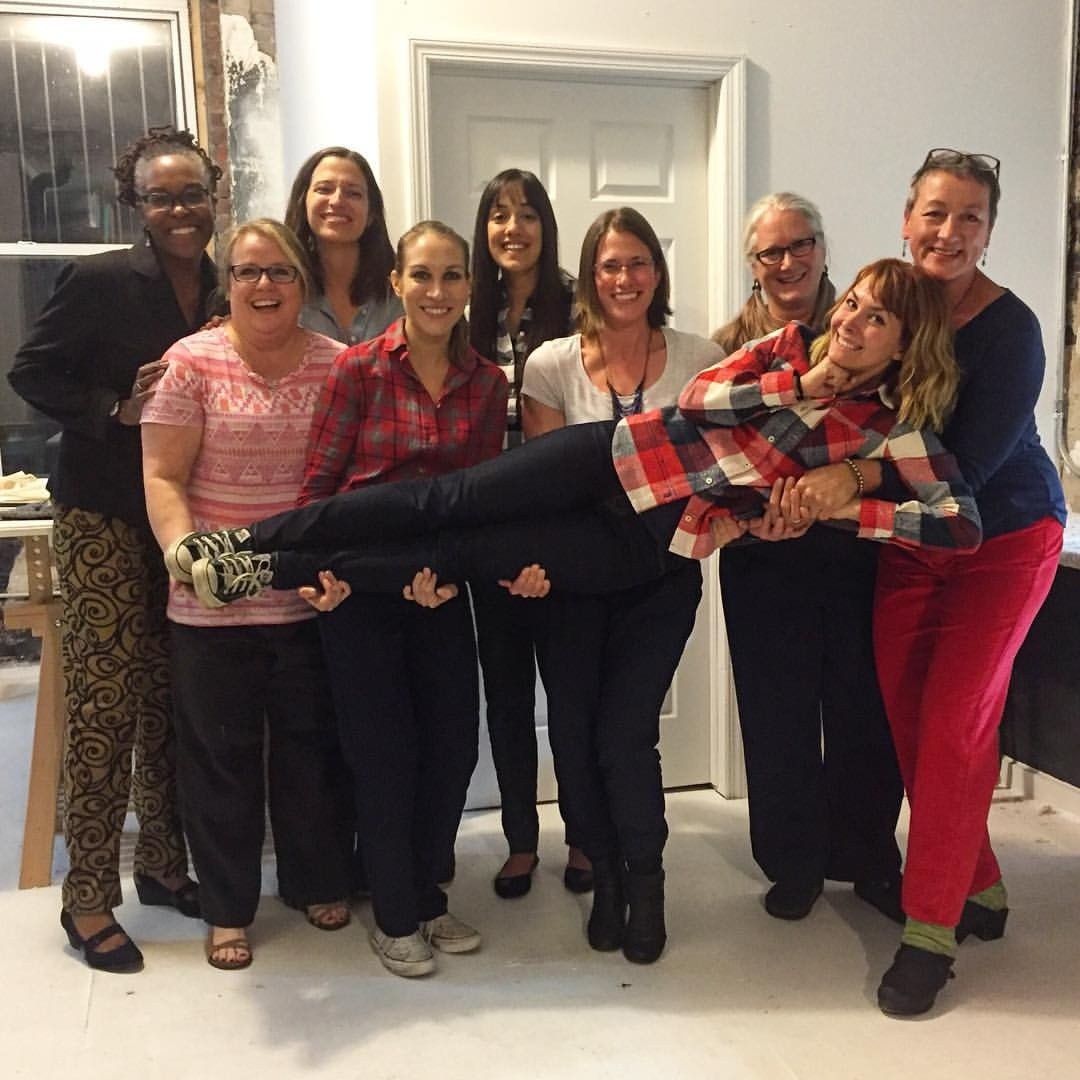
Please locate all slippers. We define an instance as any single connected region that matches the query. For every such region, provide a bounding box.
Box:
[204,925,252,970]
[305,900,352,931]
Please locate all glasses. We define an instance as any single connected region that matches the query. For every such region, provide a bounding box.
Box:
[135,186,215,212]
[913,147,1001,193]
[751,234,818,266]
[592,257,656,279]
[229,262,302,285]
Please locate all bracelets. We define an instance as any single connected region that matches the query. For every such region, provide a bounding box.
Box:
[843,457,864,497]
[795,369,804,401]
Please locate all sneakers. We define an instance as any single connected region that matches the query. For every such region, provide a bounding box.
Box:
[189,549,275,608]
[586,859,628,953]
[855,869,907,928]
[764,871,825,920]
[953,896,1012,944]
[163,526,255,584]
[371,922,436,978]
[617,869,670,964]
[417,908,483,955]
[878,944,954,1020]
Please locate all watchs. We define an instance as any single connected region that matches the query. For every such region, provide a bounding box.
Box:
[109,400,121,416]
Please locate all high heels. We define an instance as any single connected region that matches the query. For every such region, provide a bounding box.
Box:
[133,869,203,914]
[59,907,144,972]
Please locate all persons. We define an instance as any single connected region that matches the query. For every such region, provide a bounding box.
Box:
[164,258,984,607]
[11,127,231,972]
[297,219,507,976]
[141,217,352,972]
[205,145,402,346]
[465,169,593,899]
[715,193,906,927]
[766,153,1068,1018]
[522,208,729,963]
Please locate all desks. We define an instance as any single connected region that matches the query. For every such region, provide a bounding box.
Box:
[0,505,68,892]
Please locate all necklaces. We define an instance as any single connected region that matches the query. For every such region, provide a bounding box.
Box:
[768,306,813,324]
[952,267,978,310]
[594,329,653,420]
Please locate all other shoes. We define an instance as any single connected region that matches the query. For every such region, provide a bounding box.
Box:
[563,856,594,893]
[495,852,539,900]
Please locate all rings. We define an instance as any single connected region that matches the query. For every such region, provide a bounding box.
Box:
[136,383,144,393]
[791,519,801,525]
